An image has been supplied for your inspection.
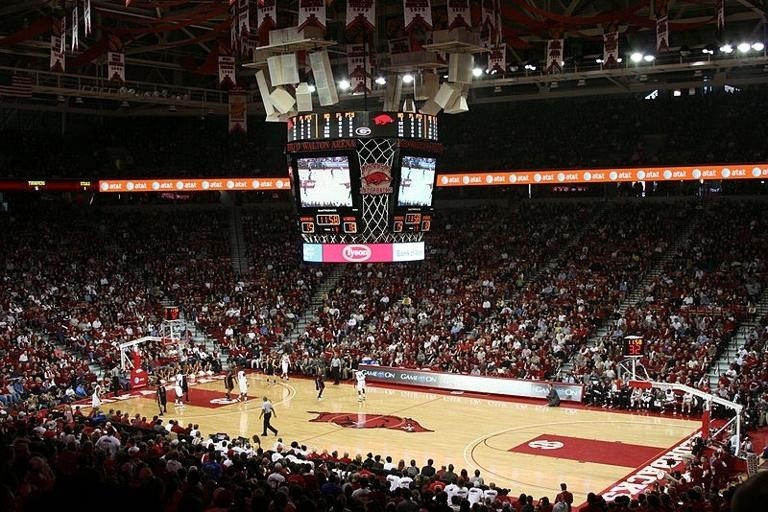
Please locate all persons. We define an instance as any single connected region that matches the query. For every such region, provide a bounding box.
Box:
[397,156,436,206]
[297,156,352,207]
